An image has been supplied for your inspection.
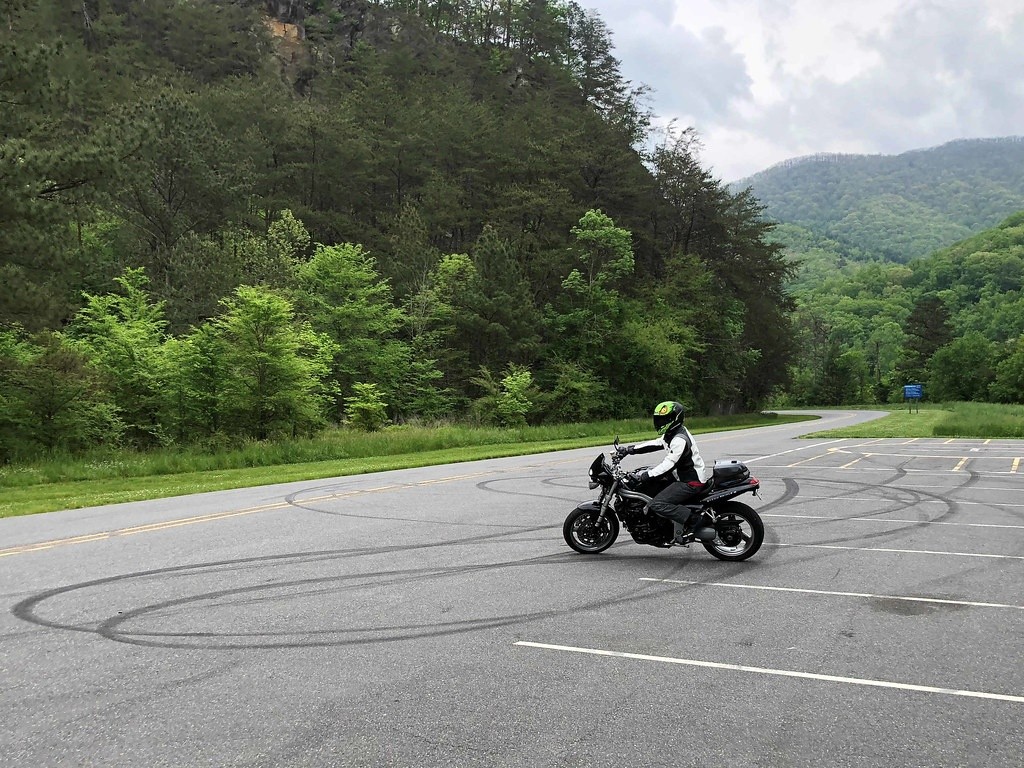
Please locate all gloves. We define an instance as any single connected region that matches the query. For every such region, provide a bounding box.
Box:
[617,445,635,460]
[628,471,649,488]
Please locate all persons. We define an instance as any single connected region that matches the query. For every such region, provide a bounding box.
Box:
[617,401,706,538]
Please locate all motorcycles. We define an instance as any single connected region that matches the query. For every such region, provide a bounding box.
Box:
[563,436,765,561]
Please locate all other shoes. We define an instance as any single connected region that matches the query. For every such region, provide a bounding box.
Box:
[681,514,700,538]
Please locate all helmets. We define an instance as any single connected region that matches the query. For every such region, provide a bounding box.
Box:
[653,401,684,435]
